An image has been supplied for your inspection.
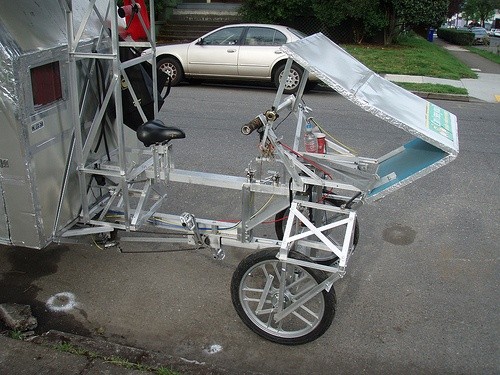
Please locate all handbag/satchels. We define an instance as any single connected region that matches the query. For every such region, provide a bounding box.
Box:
[104,30,171,130]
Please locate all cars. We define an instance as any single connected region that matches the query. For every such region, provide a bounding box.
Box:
[494,28,500,36]
[490,29,495,35]
[142,24,326,94]
[470,26,489,44]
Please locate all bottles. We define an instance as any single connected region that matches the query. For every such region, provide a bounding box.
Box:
[305,123,318,151]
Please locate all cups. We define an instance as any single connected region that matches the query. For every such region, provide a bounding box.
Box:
[307,132,325,153]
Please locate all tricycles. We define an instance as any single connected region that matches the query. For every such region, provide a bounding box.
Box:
[0,0,460,347]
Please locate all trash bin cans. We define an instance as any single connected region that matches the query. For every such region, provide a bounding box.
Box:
[422,25,429,40]
[428,27,435,41]
